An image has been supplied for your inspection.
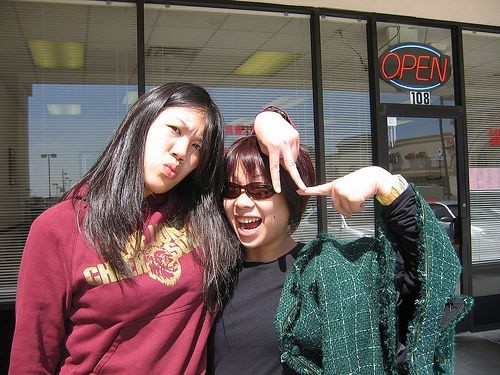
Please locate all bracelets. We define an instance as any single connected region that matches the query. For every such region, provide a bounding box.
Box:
[375,173,408,207]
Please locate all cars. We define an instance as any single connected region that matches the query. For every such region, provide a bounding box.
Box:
[424,198,500,260]
[291,200,375,244]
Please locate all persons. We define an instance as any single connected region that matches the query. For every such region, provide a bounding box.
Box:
[205,135,463,375]
[8,81,308,375]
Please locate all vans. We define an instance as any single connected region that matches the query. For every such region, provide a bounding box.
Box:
[389,136,458,199]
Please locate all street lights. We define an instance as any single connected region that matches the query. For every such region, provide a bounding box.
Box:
[52,166,72,196]
[40,152,57,198]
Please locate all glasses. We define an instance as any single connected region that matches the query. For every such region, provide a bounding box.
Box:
[220,181,275,199]
